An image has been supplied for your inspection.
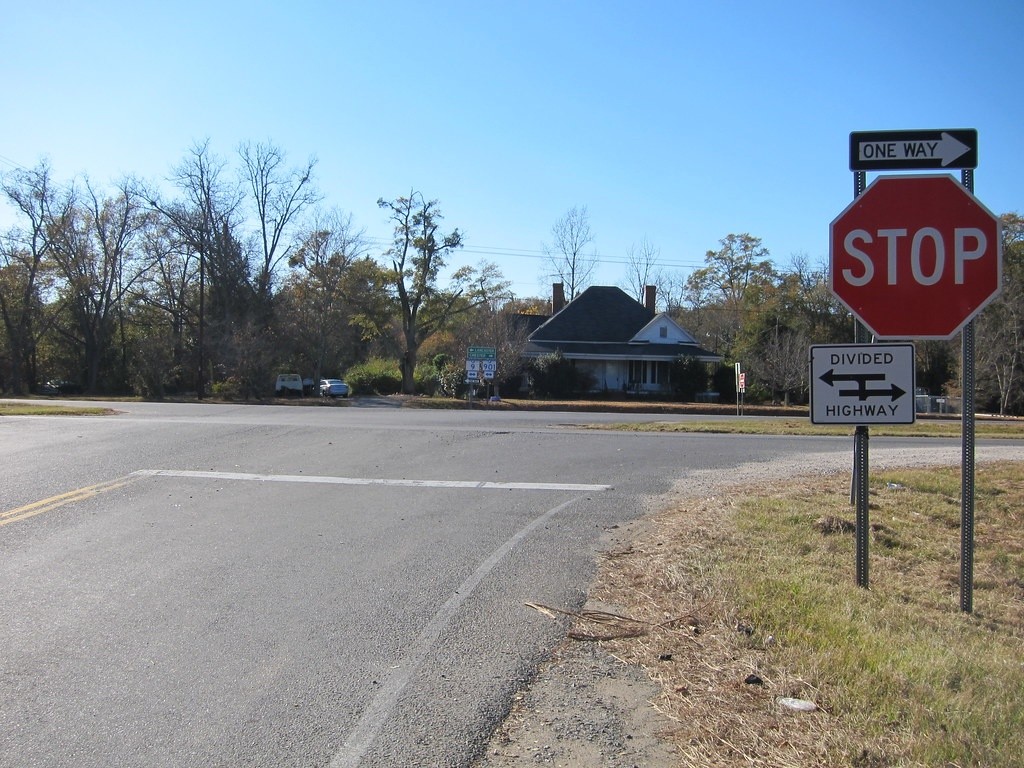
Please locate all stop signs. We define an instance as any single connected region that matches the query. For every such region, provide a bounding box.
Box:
[824,172,1005,340]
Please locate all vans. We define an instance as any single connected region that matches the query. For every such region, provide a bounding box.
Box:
[275,374,305,398]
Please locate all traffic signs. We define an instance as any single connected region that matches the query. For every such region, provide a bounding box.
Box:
[808,340,920,425]
[846,127,981,172]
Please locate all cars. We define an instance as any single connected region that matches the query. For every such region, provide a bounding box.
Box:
[312,379,350,398]
